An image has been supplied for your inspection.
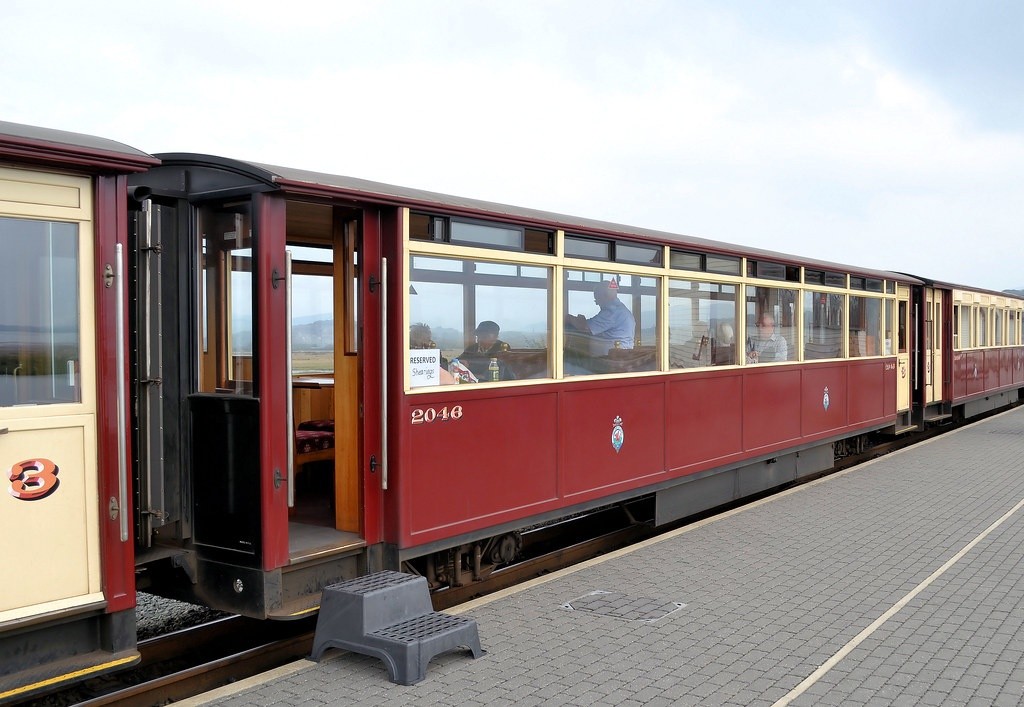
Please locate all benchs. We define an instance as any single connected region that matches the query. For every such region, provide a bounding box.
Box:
[501,339,854,374]
[224,379,336,465]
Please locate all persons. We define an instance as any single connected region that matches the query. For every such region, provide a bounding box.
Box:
[449,321,511,383]
[409,322,448,370]
[745,312,788,363]
[564,279,636,358]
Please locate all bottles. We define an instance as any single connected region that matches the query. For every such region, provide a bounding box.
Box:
[488,357,499,382]
[451,359,460,385]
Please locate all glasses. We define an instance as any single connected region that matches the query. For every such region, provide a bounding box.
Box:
[756,324,767,327]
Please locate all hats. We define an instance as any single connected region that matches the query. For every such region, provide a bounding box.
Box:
[471,321,500,335]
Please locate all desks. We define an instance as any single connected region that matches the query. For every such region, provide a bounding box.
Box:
[292,378,334,389]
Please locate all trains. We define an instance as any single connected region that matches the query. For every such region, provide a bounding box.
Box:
[0,118,1023,704]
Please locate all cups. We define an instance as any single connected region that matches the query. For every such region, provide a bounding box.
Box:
[885,339,891,355]
